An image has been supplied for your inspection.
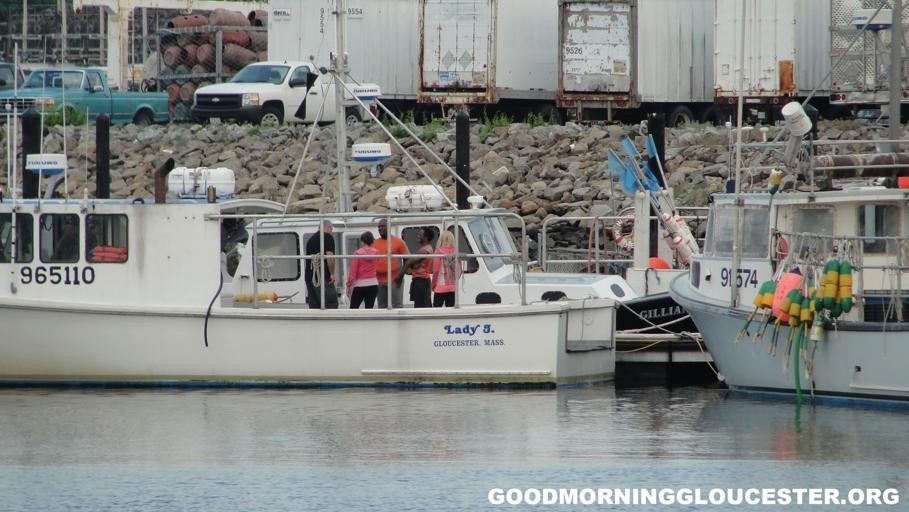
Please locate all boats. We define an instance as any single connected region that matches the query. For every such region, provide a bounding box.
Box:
[0,0,628,395]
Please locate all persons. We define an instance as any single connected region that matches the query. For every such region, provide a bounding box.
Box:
[430,231,462,308]
[394,226,435,307]
[346,219,411,309]
[305,221,338,309]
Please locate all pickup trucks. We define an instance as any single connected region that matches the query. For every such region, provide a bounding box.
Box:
[187,57,382,127]
[2,61,174,126]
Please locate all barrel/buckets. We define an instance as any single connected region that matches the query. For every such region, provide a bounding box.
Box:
[782,102,812,137]
[771,273,803,323]
[139,8,268,118]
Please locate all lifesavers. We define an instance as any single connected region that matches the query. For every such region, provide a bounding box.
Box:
[612,213,635,250]
